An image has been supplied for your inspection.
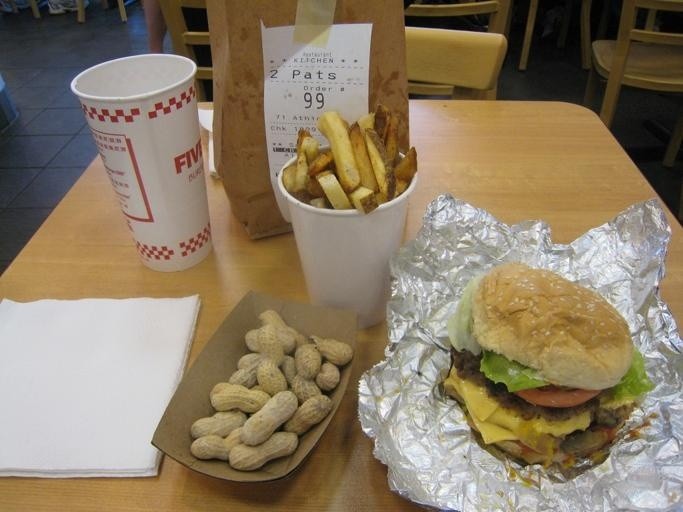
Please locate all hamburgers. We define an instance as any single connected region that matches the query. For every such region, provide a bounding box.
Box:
[437,264,658,468]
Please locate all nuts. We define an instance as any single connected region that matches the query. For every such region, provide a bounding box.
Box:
[191,310,354,472]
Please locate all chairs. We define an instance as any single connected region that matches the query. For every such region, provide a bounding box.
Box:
[159,1,213,102]
[519,0,591,70]
[585,1,683,165]
[404,1,512,98]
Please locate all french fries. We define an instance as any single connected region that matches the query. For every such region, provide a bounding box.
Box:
[281,104,417,216]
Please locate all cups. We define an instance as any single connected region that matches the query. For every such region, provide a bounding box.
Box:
[69,52,213,273]
[277,140,419,330]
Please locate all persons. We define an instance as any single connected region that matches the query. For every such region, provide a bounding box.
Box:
[141,1,168,54]
